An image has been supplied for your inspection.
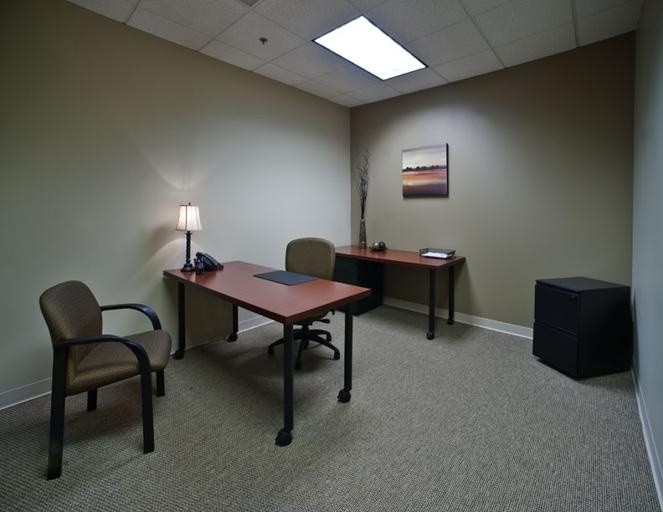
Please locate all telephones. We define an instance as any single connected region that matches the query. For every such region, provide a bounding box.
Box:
[196,252,223,270]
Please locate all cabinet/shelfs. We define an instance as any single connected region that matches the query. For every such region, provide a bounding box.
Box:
[533,276,635,378]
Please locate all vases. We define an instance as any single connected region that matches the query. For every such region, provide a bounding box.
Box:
[357,219,369,248]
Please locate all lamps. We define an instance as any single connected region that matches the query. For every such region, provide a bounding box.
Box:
[176,201,204,273]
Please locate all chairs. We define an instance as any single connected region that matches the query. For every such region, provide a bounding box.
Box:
[267,236,340,369]
[37,278,173,480]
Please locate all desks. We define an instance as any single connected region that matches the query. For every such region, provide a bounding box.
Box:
[164,259,371,446]
[330,243,466,339]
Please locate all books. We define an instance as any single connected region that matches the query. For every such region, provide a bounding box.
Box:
[421,252,453,259]
